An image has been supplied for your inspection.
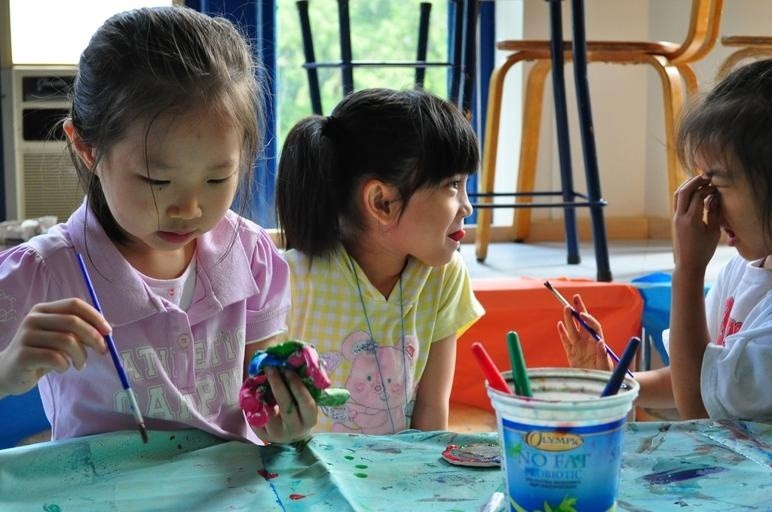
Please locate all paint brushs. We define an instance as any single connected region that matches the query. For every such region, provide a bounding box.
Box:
[545,281,635,380]
[76,252,152,445]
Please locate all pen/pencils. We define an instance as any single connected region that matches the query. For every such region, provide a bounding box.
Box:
[469,330,643,397]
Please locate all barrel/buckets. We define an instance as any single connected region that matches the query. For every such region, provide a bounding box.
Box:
[485,366,639,512]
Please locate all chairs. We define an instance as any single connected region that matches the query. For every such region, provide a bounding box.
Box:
[473,0,726,262]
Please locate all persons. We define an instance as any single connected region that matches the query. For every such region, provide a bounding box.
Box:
[277,88,486,435]
[0,7,317,444]
[556,60,772,421]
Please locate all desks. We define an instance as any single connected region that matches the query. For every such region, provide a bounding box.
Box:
[0,410,772,512]
